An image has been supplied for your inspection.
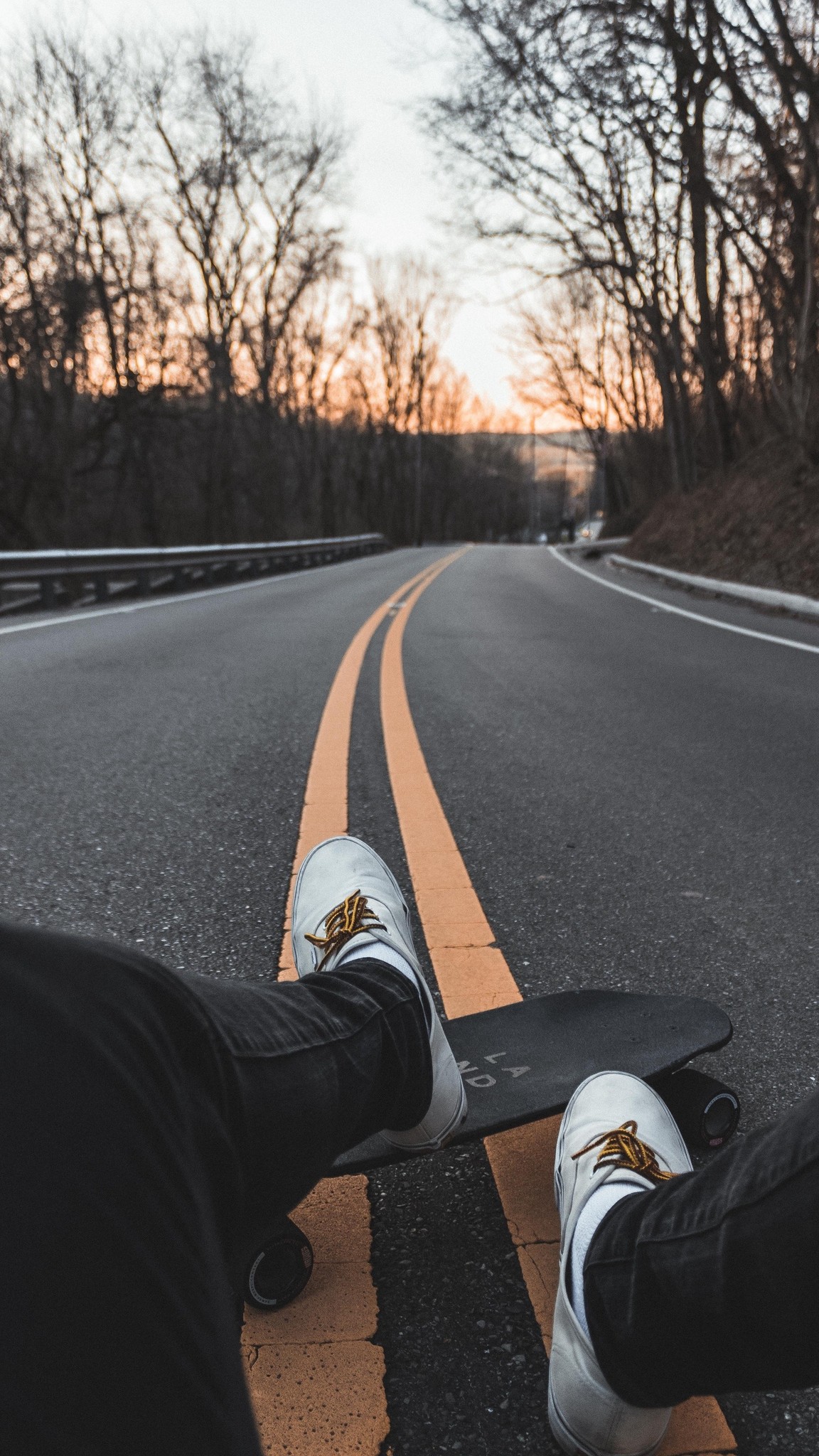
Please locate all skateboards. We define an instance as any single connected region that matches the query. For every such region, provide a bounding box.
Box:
[222,989,741,1309]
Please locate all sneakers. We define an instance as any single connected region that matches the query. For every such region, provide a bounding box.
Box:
[553,1070,696,1456]
[293,835,469,1150]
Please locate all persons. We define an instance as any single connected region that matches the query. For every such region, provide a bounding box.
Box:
[0,835,819,1456]
